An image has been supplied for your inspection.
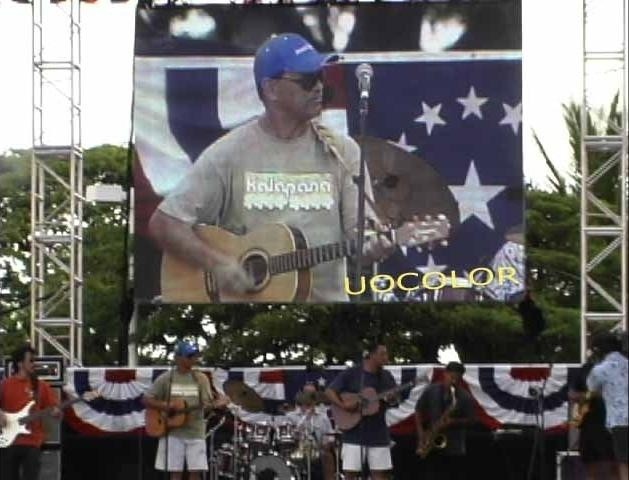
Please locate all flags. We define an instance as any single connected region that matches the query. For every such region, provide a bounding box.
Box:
[136,48,522,300]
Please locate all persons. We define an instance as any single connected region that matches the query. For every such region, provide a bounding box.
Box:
[286,380,337,480]
[566,330,620,479]
[479,185,525,300]
[414,361,479,480]
[325,341,402,480]
[585,330,629,479]
[142,339,230,479]
[147,31,398,303]
[0,346,65,480]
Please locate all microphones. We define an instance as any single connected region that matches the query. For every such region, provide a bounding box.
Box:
[355,63,374,119]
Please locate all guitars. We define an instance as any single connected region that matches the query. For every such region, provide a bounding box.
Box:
[331,376,430,431]
[160,213,453,304]
[145,395,231,437]
[0,389,103,449]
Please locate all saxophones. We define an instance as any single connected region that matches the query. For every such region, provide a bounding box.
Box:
[415,385,458,458]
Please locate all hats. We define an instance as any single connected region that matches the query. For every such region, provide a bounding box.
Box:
[253,31,340,88]
[175,341,199,357]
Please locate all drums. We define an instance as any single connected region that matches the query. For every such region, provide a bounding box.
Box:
[249,422,273,450]
[273,424,298,451]
[404,275,496,302]
[243,455,291,480]
[216,443,250,478]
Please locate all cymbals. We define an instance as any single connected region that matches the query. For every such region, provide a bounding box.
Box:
[297,393,328,404]
[223,378,263,411]
[357,137,459,244]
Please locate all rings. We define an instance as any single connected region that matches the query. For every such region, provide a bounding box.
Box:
[232,282,241,290]
